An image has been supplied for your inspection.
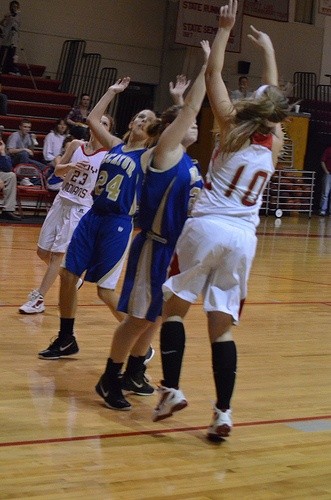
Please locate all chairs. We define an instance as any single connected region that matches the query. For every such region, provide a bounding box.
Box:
[16,165,59,218]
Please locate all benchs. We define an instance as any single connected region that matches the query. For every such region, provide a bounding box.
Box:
[0,62,78,156]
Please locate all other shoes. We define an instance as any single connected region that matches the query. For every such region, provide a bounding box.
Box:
[0,211,21,221]
[20,178,34,186]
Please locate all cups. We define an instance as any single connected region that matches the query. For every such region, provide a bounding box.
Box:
[295,105,300,113]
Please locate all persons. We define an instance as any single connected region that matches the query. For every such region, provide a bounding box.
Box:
[66,94,92,141]
[319,146,331,216]
[18,114,114,314]
[0,93,8,115]
[95,40,211,411]
[0,0,24,76]
[36,74,191,366]
[7,119,45,187]
[42,119,75,191]
[231,76,252,98]
[152,0,300,442]
[0,139,21,221]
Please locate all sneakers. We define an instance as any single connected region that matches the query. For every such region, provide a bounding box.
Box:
[75,278,82,292]
[150,380,188,422]
[38,331,80,359]
[206,401,232,439]
[95,374,132,409]
[122,366,155,396]
[18,287,45,314]
[143,345,154,365]
